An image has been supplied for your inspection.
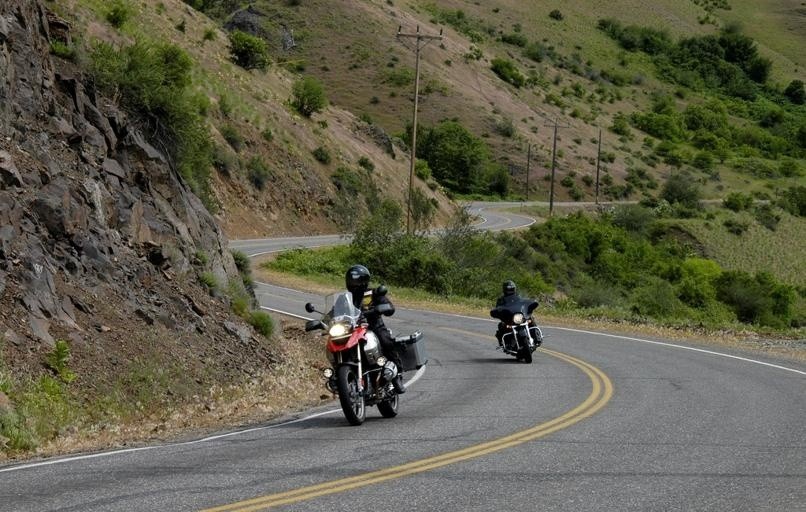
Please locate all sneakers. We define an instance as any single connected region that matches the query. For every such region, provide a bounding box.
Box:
[392,373,407,394]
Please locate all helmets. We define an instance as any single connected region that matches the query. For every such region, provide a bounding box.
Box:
[502,279,517,296]
[345,264,372,291]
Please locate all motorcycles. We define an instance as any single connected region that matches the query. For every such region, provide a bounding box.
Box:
[303,285,408,426]
[488,294,546,368]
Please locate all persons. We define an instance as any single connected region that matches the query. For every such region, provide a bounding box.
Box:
[321,265,406,393]
[495,280,543,350]
